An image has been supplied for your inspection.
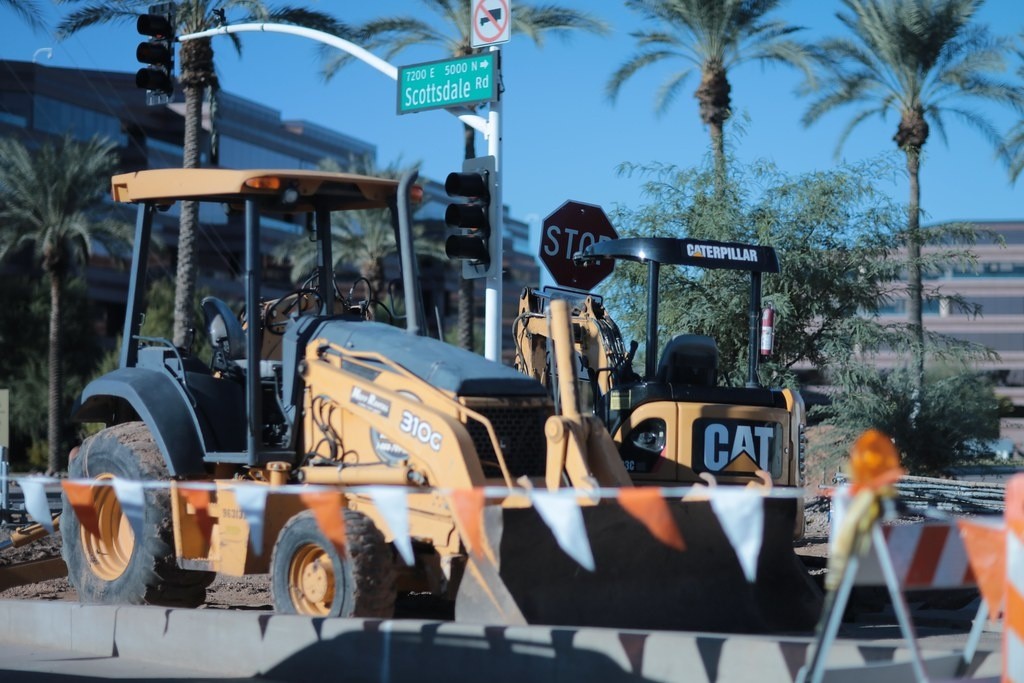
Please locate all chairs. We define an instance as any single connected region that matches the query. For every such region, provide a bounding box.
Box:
[658,334,720,387]
[202,296,282,390]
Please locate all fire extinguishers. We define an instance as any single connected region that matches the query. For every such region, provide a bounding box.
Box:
[760,300,775,355]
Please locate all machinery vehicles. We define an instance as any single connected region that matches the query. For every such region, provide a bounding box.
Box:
[58,161,824,630]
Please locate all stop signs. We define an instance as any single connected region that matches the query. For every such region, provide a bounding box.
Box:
[539,200,619,292]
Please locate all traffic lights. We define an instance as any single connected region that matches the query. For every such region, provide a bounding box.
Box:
[136,2,177,106]
[441,154,498,279]
